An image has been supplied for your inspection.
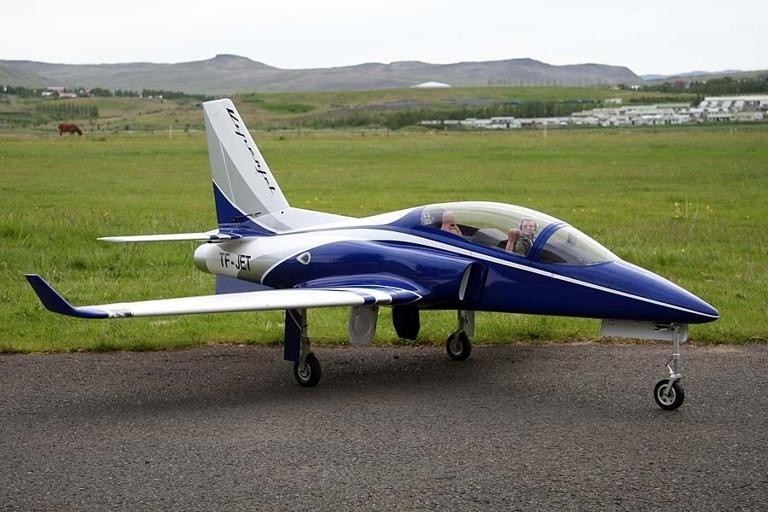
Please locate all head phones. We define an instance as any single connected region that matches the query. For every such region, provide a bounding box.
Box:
[520,218,538,231]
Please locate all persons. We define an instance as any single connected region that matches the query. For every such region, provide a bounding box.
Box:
[514,218,537,257]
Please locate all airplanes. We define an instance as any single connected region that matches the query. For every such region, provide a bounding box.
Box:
[25,97,721,411]
